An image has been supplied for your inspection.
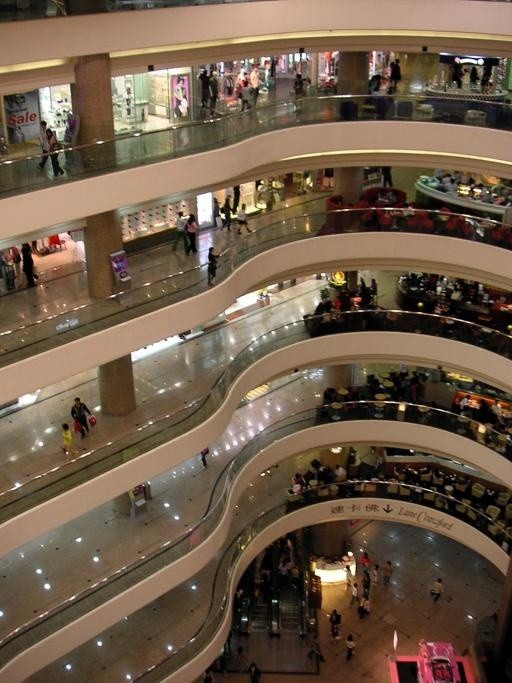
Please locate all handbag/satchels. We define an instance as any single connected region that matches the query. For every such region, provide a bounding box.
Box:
[50,143,63,152]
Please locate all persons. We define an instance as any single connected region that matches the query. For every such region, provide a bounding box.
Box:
[366,54,493,120]
[171,211,190,252]
[197,60,272,118]
[220,194,235,230]
[431,576,445,604]
[383,559,393,586]
[232,183,242,214]
[236,203,252,234]
[292,464,511,535]
[341,538,353,556]
[324,370,511,461]
[174,75,190,119]
[36,121,51,171]
[201,448,210,469]
[18,242,40,290]
[203,550,381,683]
[294,72,305,108]
[315,272,511,336]
[213,197,224,231]
[184,215,200,256]
[207,247,220,286]
[72,395,93,438]
[45,128,66,179]
[382,166,512,241]
[61,422,79,460]
[287,526,315,555]
